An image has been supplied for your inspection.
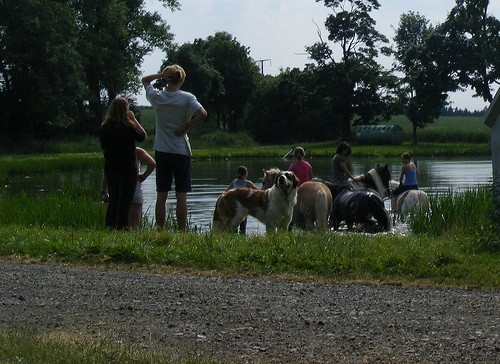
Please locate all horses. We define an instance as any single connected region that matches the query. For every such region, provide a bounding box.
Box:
[262,164,429,233]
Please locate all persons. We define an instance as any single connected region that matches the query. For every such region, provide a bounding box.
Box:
[98,97,148,231]
[142,64,207,233]
[330,142,359,231]
[225,166,259,235]
[389,153,419,214]
[288,147,313,188]
[100,147,157,233]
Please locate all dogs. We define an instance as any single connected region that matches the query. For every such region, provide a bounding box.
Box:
[212,171,300,234]
